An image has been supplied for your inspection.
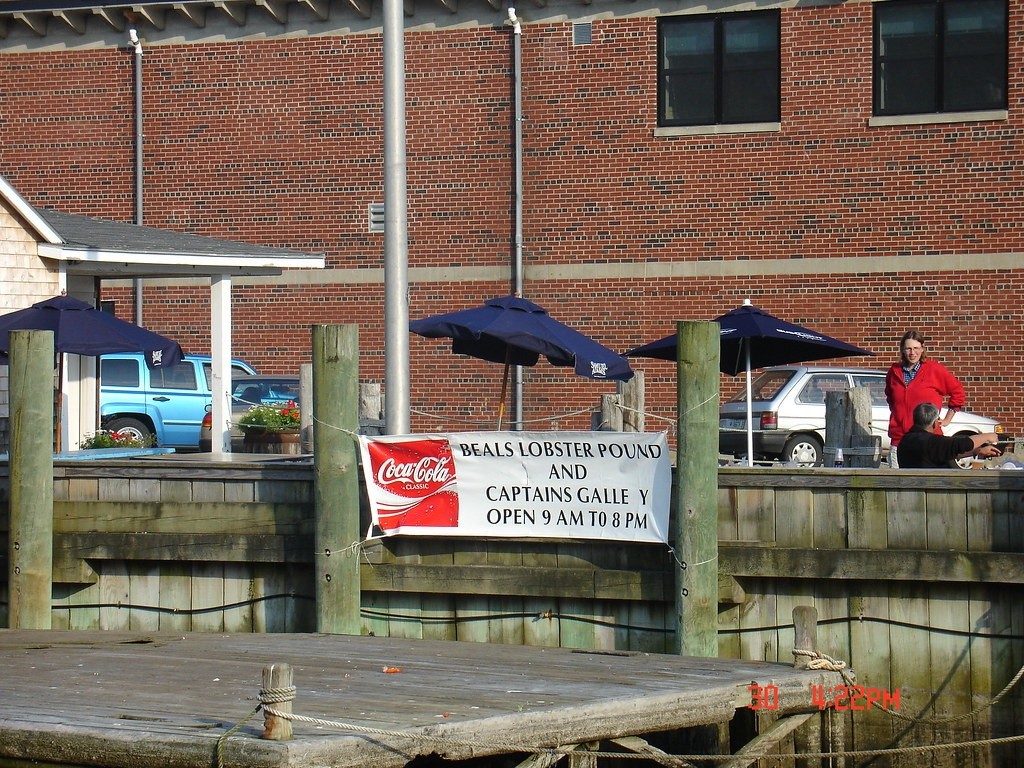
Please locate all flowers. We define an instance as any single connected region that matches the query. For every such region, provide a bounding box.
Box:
[235,398,302,435]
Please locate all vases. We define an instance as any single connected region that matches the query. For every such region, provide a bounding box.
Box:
[241,431,303,455]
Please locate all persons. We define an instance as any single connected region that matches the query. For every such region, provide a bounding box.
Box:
[884,331,1007,467]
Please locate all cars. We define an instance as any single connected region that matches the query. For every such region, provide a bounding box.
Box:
[718,365,1011,472]
[104,353,302,457]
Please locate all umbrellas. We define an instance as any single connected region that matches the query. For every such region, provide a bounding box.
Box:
[622,303,874,464]
[408,293,634,431]
[0,292,186,452]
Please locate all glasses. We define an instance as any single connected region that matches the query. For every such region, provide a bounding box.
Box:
[902,346,922,352]
